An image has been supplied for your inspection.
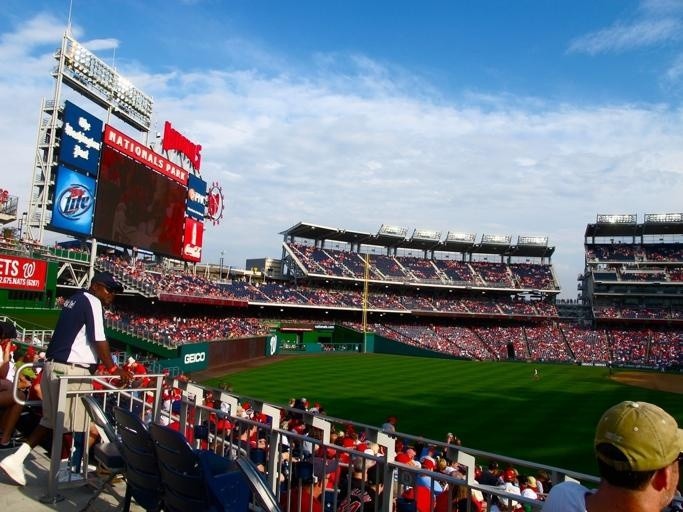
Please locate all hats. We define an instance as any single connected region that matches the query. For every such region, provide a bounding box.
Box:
[526,476,537,488]
[343,439,436,470]
[327,448,338,457]
[91,271,114,286]
[216,420,236,430]
[593,401,682,473]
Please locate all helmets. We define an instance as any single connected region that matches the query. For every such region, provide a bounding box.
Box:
[504,469,515,481]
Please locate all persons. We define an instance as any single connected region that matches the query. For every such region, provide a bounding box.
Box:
[541,400,682,512]
[541,245,682,376]
[0,237,541,511]
[608,365,615,380]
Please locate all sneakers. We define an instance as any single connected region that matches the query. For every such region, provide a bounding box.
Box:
[0,454,26,486]
[11,434,24,441]
[0,439,21,452]
[55,468,81,482]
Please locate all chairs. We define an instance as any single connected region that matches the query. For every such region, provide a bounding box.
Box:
[97,227,683,370]
[79,393,283,511]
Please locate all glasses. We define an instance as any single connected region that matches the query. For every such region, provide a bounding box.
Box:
[97,283,117,294]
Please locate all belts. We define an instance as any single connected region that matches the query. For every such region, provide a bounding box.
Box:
[45,357,87,369]
